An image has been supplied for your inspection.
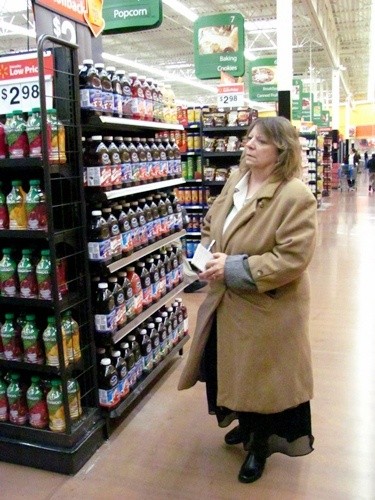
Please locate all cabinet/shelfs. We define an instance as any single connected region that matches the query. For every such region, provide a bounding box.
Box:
[1,36,343,474]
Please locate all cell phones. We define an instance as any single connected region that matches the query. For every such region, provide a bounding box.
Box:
[189,261,201,273]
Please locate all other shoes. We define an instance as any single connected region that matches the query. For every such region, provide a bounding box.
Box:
[225,425,266,483]
[183,279,208,294]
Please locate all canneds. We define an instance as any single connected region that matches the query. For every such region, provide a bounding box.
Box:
[305,139,316,194]
[175,106,210,258]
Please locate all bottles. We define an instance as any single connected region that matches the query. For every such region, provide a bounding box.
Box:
[61,311,82,364]
[0,376,8,422]
[42,316,69,369]
[63,377,82,420]
[0,107,66,164]
[46,380,66,432]
[81,134,184,191]
[79,58,180,124]
[0,313,22,362]
[26,180,48,230]
[6,180,27,230]
[0,185,7,230]
[36,250,62,301]
[7,374,27,425]
[0,248,17,298]
[21,315,44,366]
[88,192,183,267]
[26,376,49,429]
[98,298,188,411]
[17,249,37,299]
[94,243,184,336]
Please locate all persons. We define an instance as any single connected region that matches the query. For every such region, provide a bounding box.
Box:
[175,116,317,482]
[343,149,375,193]
